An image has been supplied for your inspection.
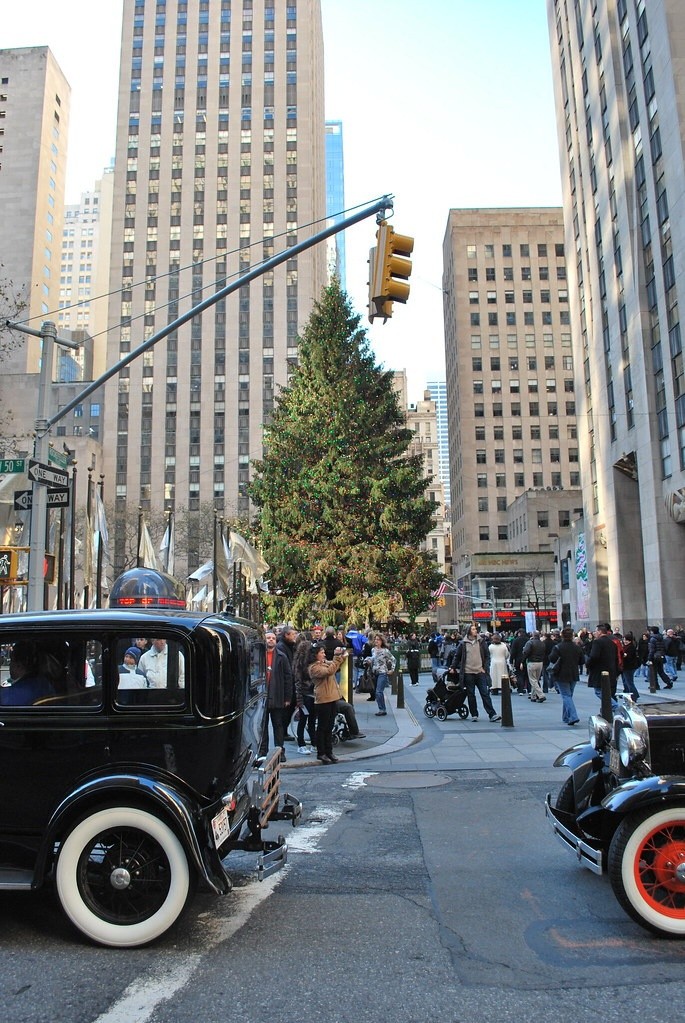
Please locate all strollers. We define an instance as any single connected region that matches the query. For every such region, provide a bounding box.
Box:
[423,669,469,721]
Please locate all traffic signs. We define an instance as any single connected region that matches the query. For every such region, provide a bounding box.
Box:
[0,459,25,474]
[27,459,69,487]
[49,445,68,468]
[14,487,70,510]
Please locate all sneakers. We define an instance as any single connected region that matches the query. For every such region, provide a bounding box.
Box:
[489,715,501,721]
[471,716,478,722]
[311,746,317,752]
[350,732,366,739]
[298,746,311,754]
[281,747,286,762]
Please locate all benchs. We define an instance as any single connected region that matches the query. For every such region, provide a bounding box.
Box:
[33,685,102,706]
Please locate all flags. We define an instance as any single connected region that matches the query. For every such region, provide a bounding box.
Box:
[428,580,447,610]
[2,468,271,613]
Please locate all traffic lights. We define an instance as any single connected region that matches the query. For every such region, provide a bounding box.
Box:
[372,224,414,304]
[0,549,18,580]
[42,554,57,583]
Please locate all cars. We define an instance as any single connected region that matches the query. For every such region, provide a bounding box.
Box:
[544,671,685,940]
[0,607,303,949]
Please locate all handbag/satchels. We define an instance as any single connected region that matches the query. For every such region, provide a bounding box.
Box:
[546,645,561,675]
[358,664,377,693]
[653,653,667,664]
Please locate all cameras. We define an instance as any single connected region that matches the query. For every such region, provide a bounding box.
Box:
[340,647,346,651]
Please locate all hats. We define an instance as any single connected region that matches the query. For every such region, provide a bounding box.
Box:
[466,624,472,634]
[125,647,142,661]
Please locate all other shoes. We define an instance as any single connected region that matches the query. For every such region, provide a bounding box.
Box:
[531,699,536,702]
[537,697,546,702]
[663,682,673,689]
[409,683,418,687]
[672,675,678,681]
[367,697,375,701]
[285,735,295,741]
[519,693,523,696]
[529,692,531,695]
[375,712,387,716]
[568,719,579,726]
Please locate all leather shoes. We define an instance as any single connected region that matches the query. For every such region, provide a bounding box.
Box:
[328,754,338,761]
[317,755,331,762]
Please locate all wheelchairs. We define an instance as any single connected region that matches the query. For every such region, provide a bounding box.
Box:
[291,700,350,747]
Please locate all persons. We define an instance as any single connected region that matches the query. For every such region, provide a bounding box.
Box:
[480,628,562,703]
[118,638,185,690]
[312,624,376,702]
[257,626,317,762]
[384,632,429,676]
[548,627,586,726]
[428,629,463,680]
[406,645,420,687]
[449,625,501,722]
[308,643,367,762]
[364,633,397,716]
[586,624,618,716]
[0,641,86,705]
[573,623,685,701]
